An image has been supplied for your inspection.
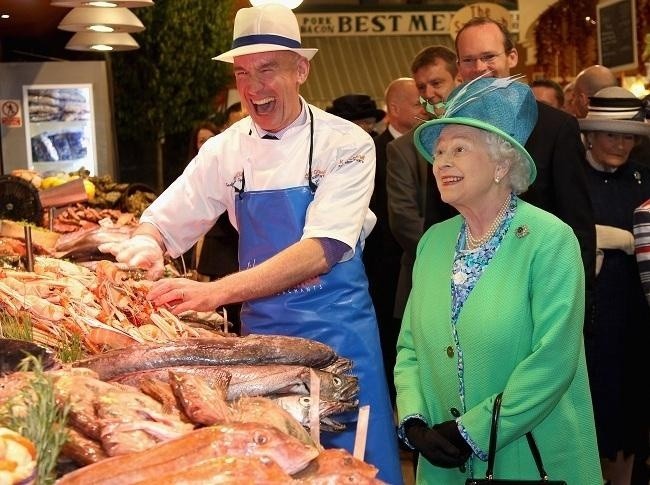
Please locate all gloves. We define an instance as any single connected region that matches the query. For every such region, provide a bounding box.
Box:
[595,224,635,255]
[404,418,472,468]
[98,236,165,274]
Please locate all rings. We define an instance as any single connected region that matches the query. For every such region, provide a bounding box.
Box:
[180,288,184,299]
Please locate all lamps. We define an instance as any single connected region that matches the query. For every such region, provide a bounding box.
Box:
[64,30,141,52]
[54,7,147,34]
[49,0,156,7]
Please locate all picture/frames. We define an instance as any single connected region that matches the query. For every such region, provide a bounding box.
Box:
[21,81,98,178]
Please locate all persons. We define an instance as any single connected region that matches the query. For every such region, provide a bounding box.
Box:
[391,75,604,485]
[76,9,404,484]
[191,17,617,407]
[548,86,650,461]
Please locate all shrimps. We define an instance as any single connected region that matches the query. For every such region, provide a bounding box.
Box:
[1,255,233,357]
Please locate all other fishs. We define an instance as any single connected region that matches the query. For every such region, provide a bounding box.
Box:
[55,225,171,271]
[0,333,391,485]
[27,88,91,123]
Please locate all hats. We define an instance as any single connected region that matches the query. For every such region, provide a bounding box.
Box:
[577,87,650,136]
[211,3,318,63]
[326,95,385,123]
[413,77,538,187]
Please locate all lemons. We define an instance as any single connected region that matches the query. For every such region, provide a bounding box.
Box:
[40,176,96,200]
